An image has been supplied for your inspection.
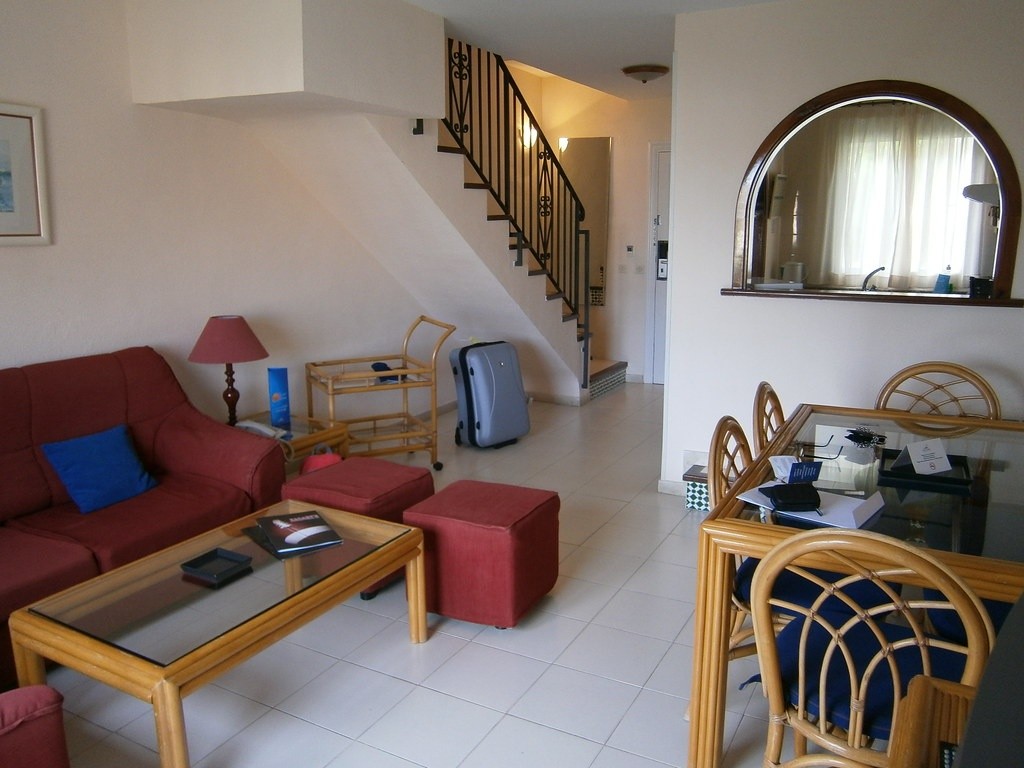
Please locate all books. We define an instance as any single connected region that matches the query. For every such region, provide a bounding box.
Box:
[240,510,345,562]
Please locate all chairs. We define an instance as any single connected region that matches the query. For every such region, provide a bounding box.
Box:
[750,527,995,768]
[875,360,1001,477]
[753,381,787,456]
[919,586,1014,652]
[708,415,902,667]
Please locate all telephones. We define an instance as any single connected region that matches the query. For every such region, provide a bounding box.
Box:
[233,419,289,440]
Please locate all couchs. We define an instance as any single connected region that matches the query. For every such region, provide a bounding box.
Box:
[0,682,67,768]
[0,345,286,688]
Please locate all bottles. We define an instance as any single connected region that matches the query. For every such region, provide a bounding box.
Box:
[946,266,954,291]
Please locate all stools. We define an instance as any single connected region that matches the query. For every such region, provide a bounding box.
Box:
[281,458,434,599]
[401,480,560,629]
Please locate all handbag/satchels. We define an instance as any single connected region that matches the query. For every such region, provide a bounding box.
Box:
[298,443,342,474]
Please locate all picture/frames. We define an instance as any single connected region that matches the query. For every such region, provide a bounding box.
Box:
[0,103,52,248]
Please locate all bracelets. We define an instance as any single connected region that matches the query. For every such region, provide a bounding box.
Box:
[854,426,878,448]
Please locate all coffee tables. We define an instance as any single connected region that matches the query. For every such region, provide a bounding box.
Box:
[8,498,427,768]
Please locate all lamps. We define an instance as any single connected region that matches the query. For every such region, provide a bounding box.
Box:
[189,314,270,425]
[622,66,669,84]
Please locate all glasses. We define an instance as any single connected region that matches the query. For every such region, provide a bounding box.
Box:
[795,435,843,463]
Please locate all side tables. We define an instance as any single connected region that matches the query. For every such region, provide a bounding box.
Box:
[237,410,350,472]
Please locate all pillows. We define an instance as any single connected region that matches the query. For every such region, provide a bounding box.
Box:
[37,428,158,512]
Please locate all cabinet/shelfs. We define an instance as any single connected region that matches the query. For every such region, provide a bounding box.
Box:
[307,314,455,464]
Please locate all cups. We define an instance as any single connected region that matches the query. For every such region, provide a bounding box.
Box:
[782,262,810,284]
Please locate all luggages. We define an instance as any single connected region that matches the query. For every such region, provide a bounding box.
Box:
[448,341,529,450]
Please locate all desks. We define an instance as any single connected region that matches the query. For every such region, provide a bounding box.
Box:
[686,402,1024,768]
